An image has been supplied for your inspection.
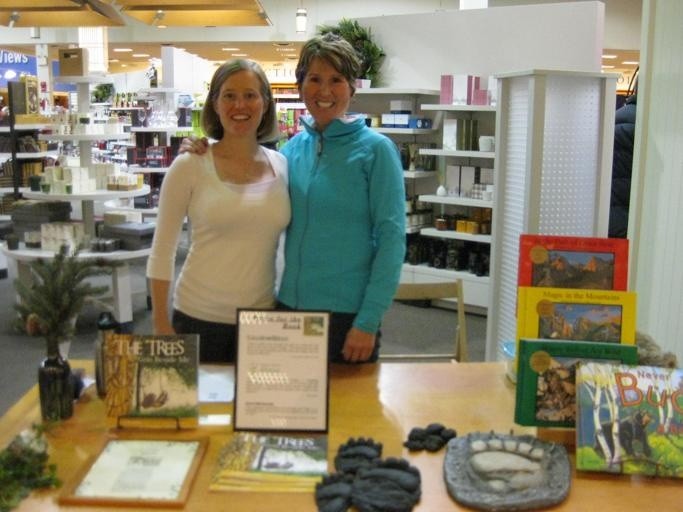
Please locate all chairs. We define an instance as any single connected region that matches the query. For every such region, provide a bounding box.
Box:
[374,278,472,365]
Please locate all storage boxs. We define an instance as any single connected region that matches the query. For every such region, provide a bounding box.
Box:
[58,48,91,75]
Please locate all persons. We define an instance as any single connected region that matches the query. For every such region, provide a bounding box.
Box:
[175,32,406,374]
[142,59,295,365]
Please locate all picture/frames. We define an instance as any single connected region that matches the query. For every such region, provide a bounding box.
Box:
[53,429,212,507]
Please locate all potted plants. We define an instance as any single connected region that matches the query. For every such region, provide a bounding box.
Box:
[317,19,387,90]
[8,239,114,424]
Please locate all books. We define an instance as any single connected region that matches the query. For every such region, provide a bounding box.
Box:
[203,429,332,498]
[511,234,639,426]
[573,361,683,479]
[104,332,199,416]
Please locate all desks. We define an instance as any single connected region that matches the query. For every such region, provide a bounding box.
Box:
[0,362,682,512]
